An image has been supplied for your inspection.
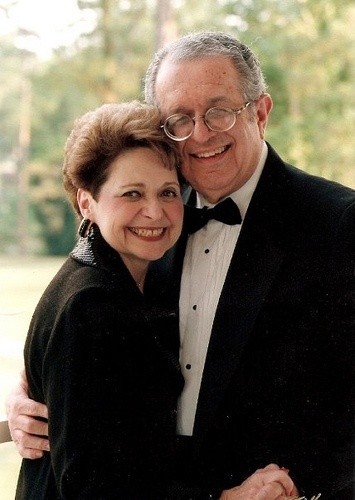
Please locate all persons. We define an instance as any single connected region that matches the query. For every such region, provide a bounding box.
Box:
[13,101,297,500]
[5,31,355,499]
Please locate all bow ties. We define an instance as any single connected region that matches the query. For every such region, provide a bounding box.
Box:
[182,197,242,234]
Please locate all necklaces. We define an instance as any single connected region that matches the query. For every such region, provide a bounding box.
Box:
[136,279,145,287]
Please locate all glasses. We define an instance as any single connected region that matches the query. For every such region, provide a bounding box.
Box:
[160,101,252,142]
